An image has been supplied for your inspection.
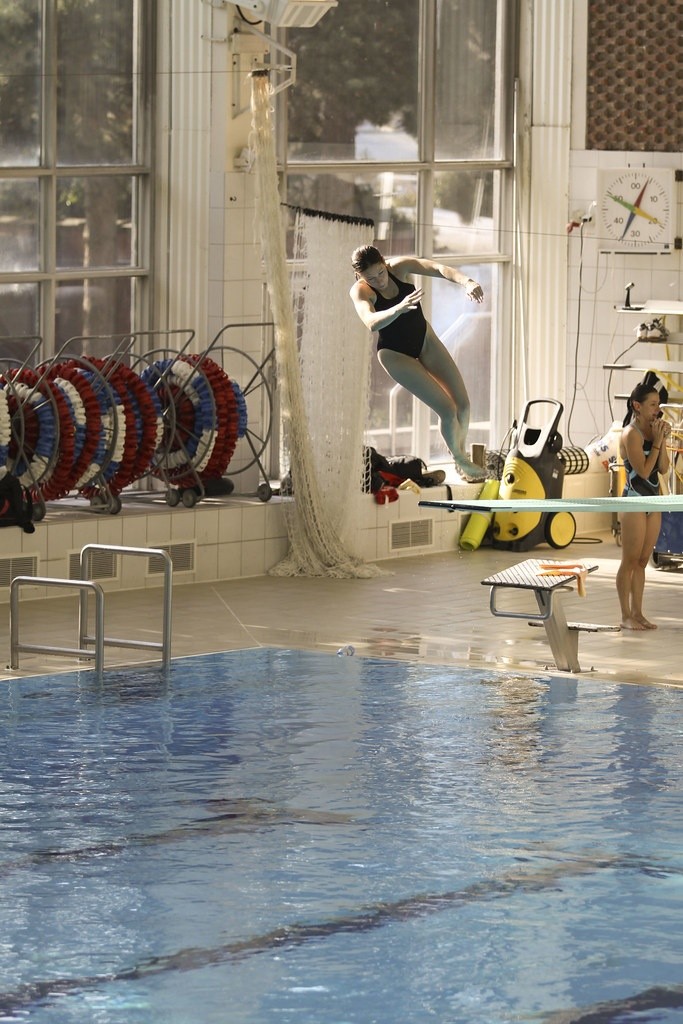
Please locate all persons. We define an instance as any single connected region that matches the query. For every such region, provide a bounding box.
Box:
[616,384,673,631]
[348,245,490,482]
